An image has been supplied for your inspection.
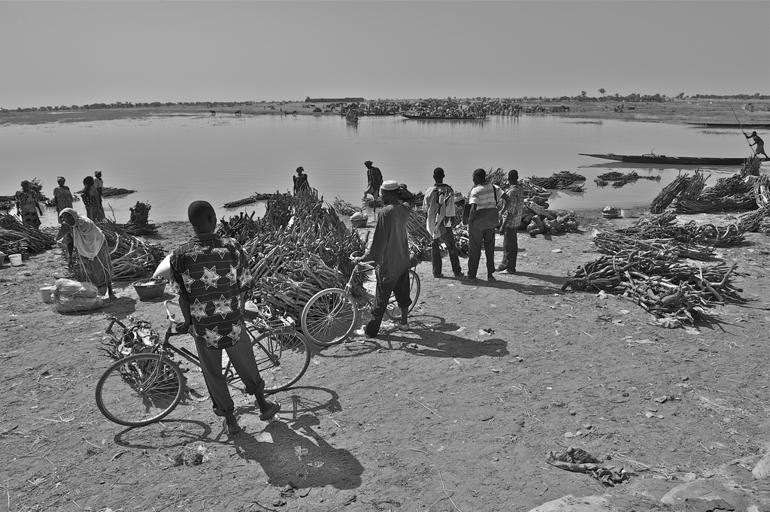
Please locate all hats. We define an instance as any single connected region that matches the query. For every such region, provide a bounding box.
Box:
[380,179,400,190]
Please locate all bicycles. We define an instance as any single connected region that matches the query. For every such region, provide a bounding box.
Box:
[301,251,418,347]
[94,298,310,424]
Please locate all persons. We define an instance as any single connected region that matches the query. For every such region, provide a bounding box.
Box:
[169,200,281,435]
[15,180,44,230]
[57,207,117,301]
[362,160,383,214]
[93,169,103,205]
[292,166,310,197]
[743,131,769,162]
[462,168,512,283]
[77,176,106,221]
[422,167,464,279]
[52,175,73,228]
[264,96,545,127]
[495,170,524,275]
[361,180,412,339]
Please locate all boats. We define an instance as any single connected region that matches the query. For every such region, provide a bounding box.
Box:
[345,109,359,124]
[579,152,770,166]
[403,113,489,119]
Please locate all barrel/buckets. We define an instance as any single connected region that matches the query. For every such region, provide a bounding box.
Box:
[8,253,22,266]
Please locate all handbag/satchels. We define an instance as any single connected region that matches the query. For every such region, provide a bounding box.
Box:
[470,208,500,238]
